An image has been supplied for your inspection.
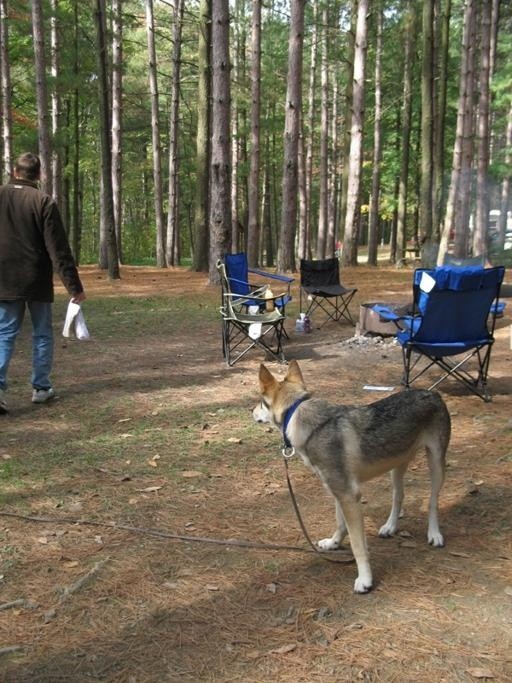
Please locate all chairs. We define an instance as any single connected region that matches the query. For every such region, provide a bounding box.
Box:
[299,258,359,327]
[371,264,505,403]
[215,252,295,366]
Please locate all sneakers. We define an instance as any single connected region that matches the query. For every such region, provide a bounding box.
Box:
[0,401,9,414]
[32,388,54,403]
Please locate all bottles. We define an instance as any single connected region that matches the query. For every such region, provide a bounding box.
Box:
[303,315,310,334]
[265,287,274,312]
[295,315,302,332]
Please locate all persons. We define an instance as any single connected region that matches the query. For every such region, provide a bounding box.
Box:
[1,152,87,414]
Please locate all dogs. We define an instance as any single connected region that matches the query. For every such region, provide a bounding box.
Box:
[250,358,451,594]
